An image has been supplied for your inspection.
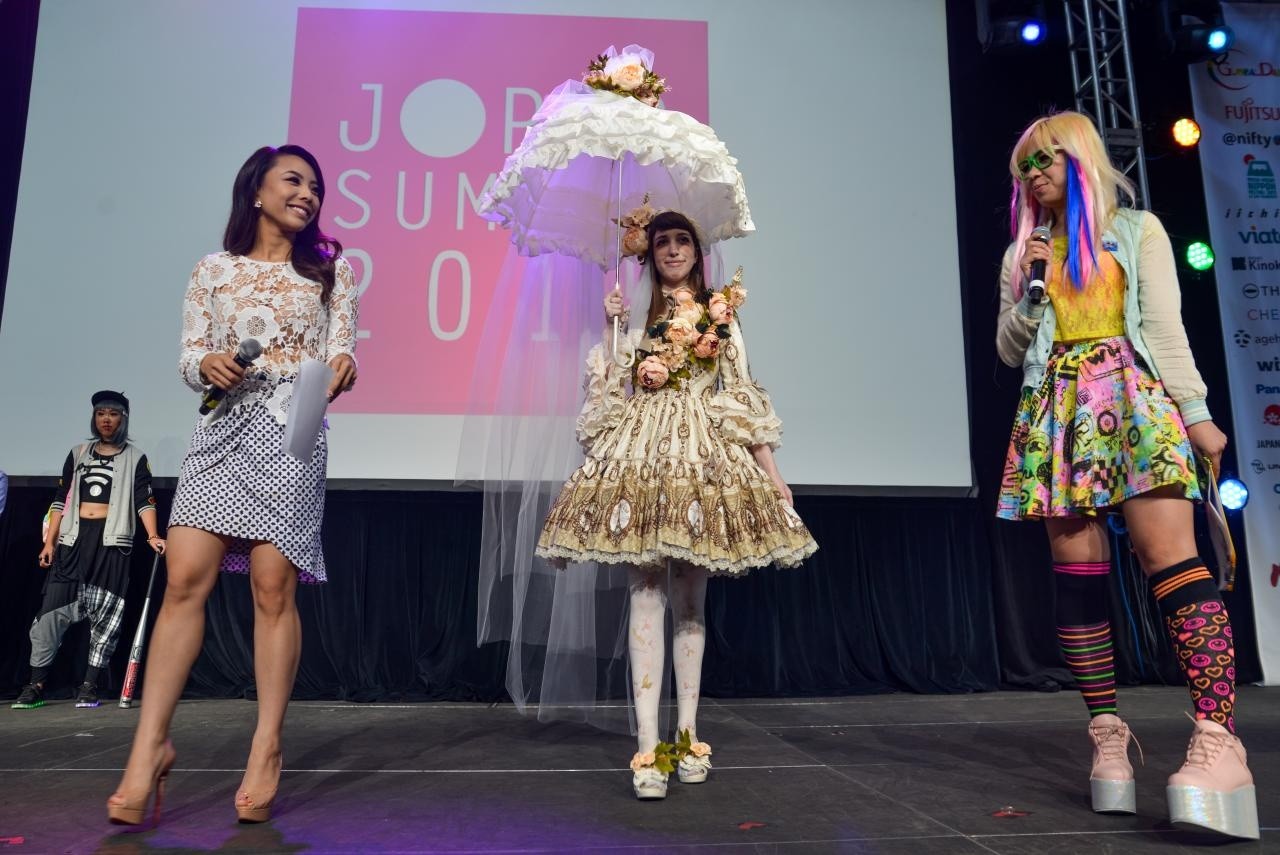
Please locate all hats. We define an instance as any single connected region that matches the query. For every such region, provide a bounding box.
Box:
[92,390,130,410]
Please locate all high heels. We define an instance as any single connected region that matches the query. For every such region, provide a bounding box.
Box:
[1165,712,1261,839]
[109,738,176,825]
[234,750,282,822]
[1088,714,1145,813]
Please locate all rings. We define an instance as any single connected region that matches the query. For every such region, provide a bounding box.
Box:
[610,297,613,303]
[348,384,352,391]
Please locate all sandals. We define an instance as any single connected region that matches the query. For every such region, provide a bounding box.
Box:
[675,727,711,783]
[630,742,674,798]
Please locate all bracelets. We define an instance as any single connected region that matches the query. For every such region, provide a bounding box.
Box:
[147,536,161,542]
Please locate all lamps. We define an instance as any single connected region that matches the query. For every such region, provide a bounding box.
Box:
[1218,467,1250,512]
[1176,240,1215,295]
[1159,23,1232,68]
[1147,116,1201,160]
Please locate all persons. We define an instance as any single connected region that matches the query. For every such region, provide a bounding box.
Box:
[995,111,1260,839]
[108,144,358,825]
[535,210,820,799]
[17,391,167,708]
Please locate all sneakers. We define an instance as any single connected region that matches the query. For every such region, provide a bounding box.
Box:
[75,685,99,707]
[11,686,46,708]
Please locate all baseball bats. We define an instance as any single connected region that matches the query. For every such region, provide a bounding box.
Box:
[118,543,164,710]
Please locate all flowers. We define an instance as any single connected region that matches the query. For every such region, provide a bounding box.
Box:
[631,264,750,391]
[578,49,672,107]
[610,190,659,257]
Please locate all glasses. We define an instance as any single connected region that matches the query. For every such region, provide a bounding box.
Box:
[1017,147,1063,180]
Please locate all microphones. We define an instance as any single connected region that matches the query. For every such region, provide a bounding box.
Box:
[199,338,261,416]
[1028,226,1051,305]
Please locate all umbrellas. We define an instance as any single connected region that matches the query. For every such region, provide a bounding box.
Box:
[476,43,756,368]
